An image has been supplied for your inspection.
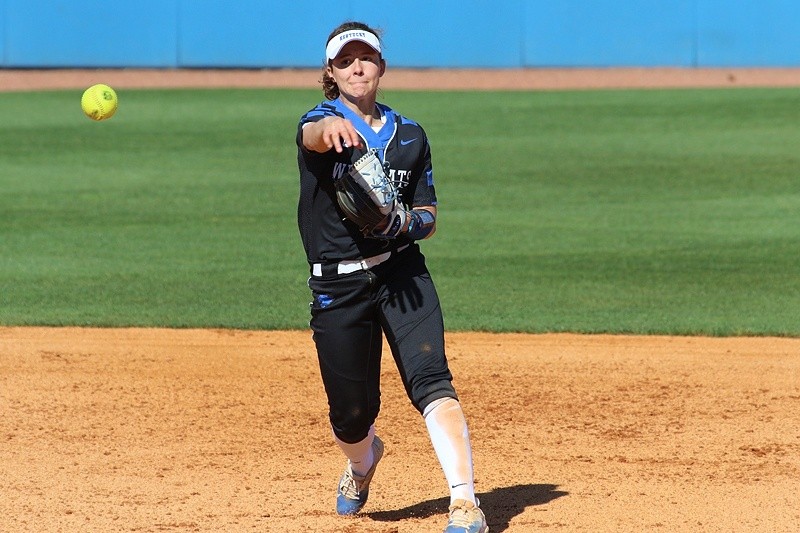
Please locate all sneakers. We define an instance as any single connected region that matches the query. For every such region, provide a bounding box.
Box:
[445,499,489,533]
[336,435,385,515]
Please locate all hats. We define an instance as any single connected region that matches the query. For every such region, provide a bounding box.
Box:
[324,30,382,64]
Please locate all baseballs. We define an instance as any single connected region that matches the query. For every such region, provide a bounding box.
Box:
[81,83,119,121]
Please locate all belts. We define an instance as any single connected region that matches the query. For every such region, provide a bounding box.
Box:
[312,245,410,277]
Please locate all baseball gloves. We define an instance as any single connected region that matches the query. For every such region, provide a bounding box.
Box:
[333,147,407,249]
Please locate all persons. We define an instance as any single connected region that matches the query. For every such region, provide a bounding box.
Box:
[296,22,489,533]
